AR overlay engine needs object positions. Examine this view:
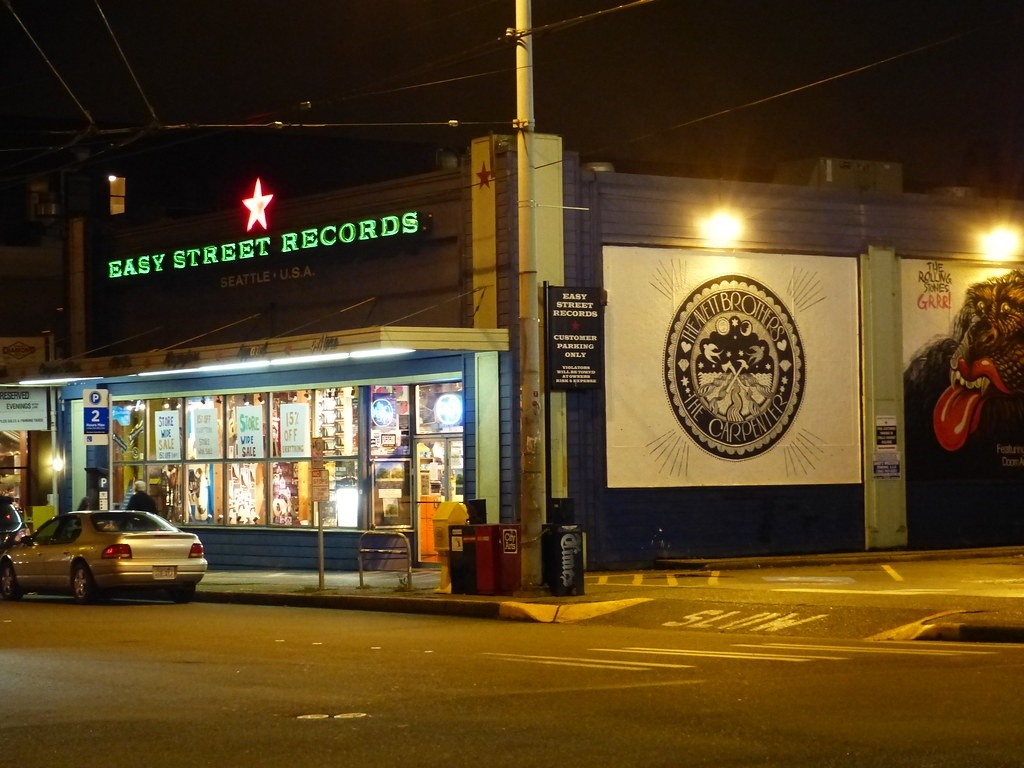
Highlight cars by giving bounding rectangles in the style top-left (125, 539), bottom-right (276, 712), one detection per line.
top-left (1, 510), bottom-right (208, 604)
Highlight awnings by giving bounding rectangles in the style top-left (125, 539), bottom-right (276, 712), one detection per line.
top-left (0, 325), bottom-right (508, 386)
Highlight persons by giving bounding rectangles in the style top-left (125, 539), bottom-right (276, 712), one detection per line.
top-left (120, 481), bottom-right (159, 532)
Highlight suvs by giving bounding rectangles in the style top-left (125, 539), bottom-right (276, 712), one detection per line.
top-left (0, 495), bottom-right (31, 600)
